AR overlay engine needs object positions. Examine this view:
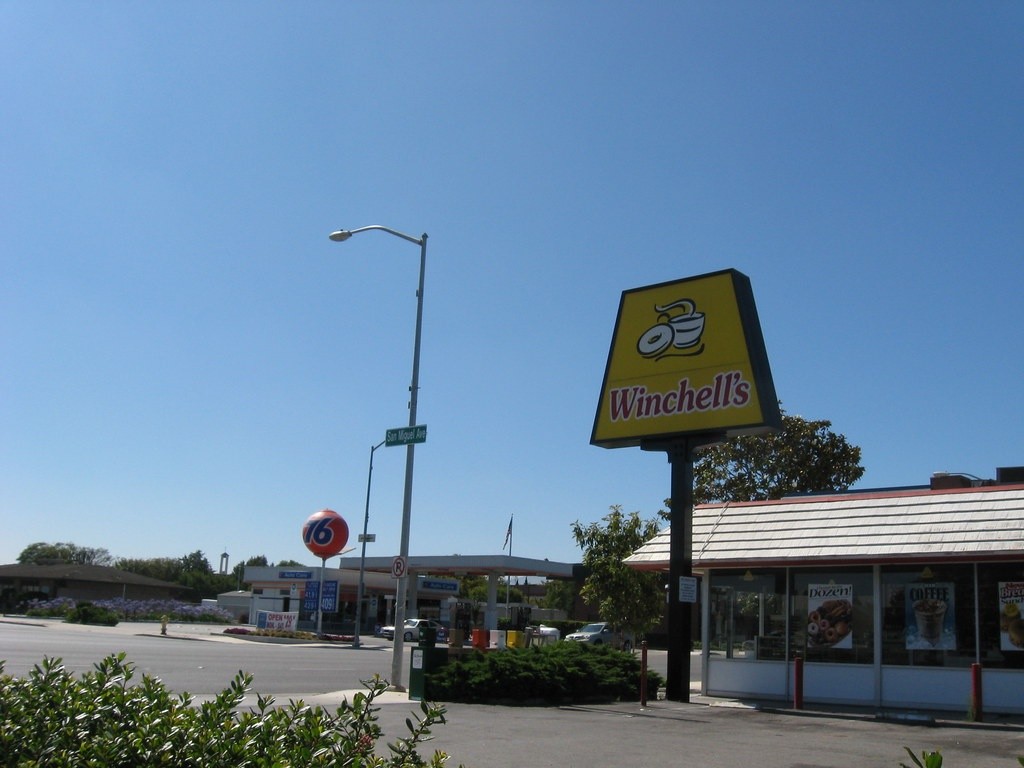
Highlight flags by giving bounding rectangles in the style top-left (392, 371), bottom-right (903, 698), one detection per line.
top-left (503, 516), bottom-right (512, 550)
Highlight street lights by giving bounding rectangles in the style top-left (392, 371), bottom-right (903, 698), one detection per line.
top-left (329, 227), bottom-right (431, 692)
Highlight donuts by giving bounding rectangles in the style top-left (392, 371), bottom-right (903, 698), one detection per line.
top-left (806, 610), bottom-right (838, 642)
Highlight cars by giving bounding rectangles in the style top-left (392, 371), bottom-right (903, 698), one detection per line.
top-left (379, 619), bottom-right (441, 643)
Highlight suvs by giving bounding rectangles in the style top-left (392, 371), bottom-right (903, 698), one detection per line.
top-left (564, 621), bottom-right (636, 654)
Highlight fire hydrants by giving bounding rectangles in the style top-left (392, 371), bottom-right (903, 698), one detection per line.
top-left (160, 615), bottom-right (169, 635)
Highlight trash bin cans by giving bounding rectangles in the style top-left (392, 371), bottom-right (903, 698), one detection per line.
top-left (472, 629), bottom-right (488, 649)
top-left (409, 645), bottom-right (449, 701)
top-left (448, 628), bottom-right (463, 647)
top-left (507, 630), bottom-right (526, 650)
top-left (418, 627), bottom-right (436, 647)
top-left (490, 630), bottom-right (506, 649)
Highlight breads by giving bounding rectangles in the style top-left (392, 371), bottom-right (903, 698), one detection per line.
top-left (1000, 602), bottom-right (1024, 648)
top-left (822, 601), bottom-right (849, 635)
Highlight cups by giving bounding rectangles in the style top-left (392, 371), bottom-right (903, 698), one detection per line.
top-left (913, 600), bottom-right (947, 646)
